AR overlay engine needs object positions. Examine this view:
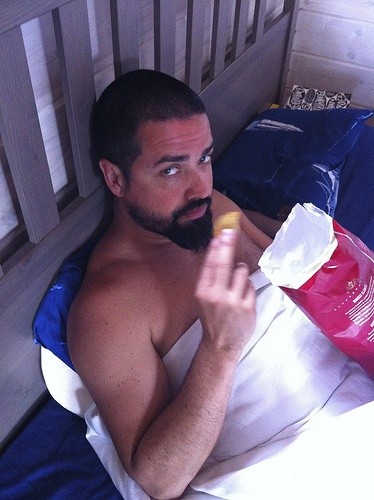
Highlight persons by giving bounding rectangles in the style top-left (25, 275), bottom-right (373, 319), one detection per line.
top-left (66, 67), bottom-right (374, 499)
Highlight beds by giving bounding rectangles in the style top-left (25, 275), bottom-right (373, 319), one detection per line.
top-left (0, 1), bottom-right (374, 499)
